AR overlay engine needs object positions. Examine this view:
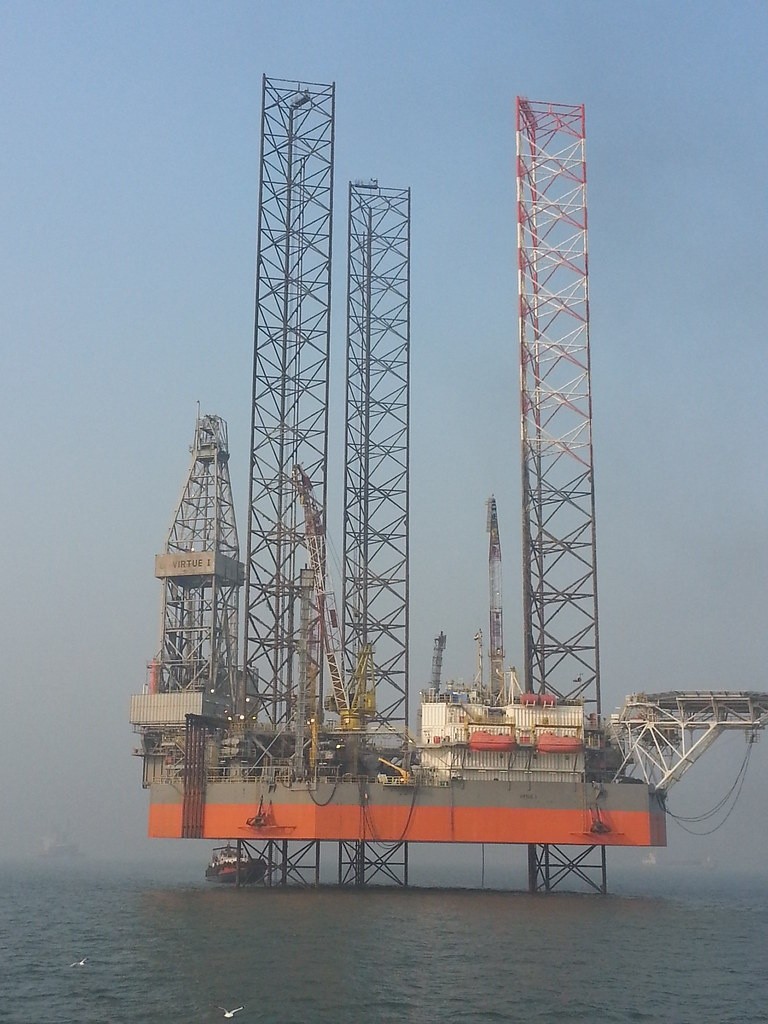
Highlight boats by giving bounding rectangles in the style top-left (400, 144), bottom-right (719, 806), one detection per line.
top-left (205, 841), bottom-right (269, 885)
